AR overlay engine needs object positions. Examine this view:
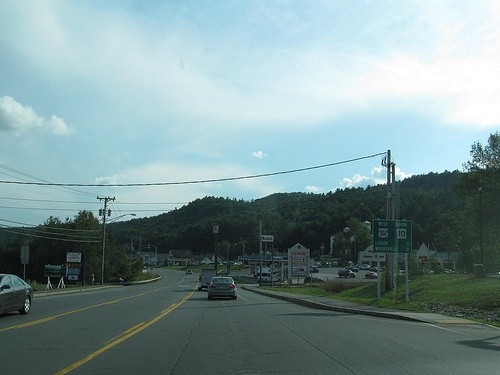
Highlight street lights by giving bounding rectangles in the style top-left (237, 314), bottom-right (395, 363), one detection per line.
top-left (101, 213), bottom-right (137, 284)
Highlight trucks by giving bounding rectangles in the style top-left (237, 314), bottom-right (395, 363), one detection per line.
top-left (338, 269), bottom-right (356, 278)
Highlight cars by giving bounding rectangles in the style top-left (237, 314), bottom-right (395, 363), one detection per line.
top-left (0, 274), bottom-right (33, 315)
top-left (198, 271), bottom-right (217, 291)
top-left (257, 273), bottom-right (280, 283)
top-left (365, 272), bottom-right (378, 279)
top-left (208, 276), bottom-right (237, 300)
